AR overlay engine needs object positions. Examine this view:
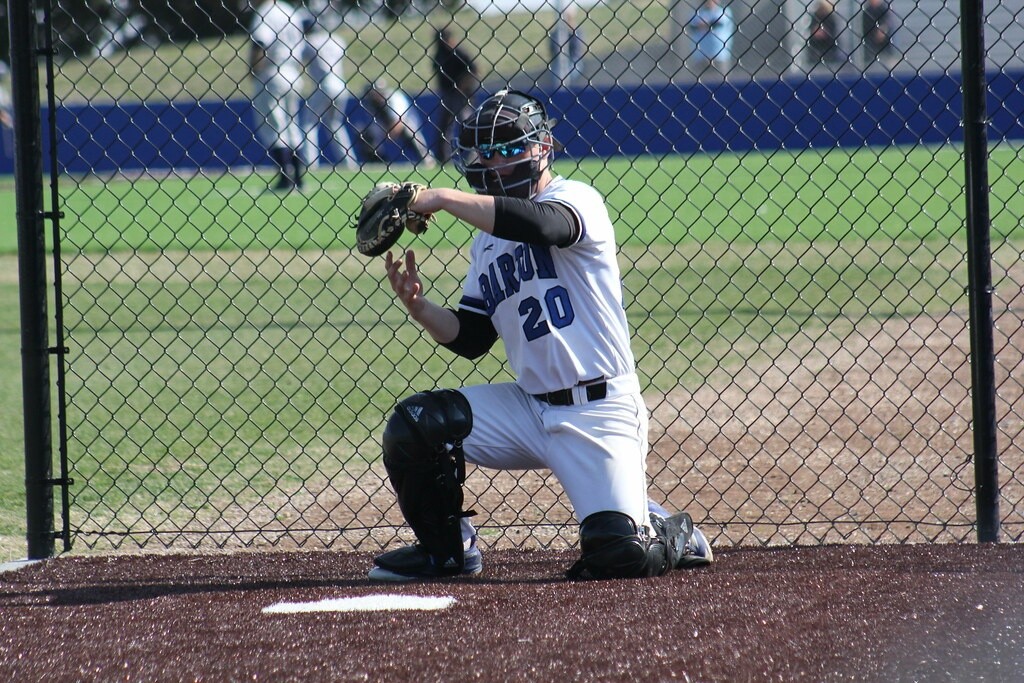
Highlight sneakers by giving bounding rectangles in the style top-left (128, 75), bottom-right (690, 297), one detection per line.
top-left (369, 544), bottom-right (483, 581)
top-left (647, 498), bottom-right (713, 568)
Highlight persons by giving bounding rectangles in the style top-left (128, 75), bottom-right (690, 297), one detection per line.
top-left (862, 0), bottom-right (901, 71)
top-left (299, 18), bottom-right (359, 171)
top-left (434, 27), bottom-right (480, 167)
top-left (549, 2), bottom-right (582, 87)
top-left (247, 0), bottom-right (307, 190)
top-left (688, 0), bottom-right (733, 82)
top-left (810, 0), bottom-right (843, 76)
top-left (363, 74), bottom-right (436, 170)
top-left (355, 89), bottom-right (712, 583)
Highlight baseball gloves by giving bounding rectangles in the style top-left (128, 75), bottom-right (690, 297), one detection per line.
top-left (348, 182), bottom-right (431, 257)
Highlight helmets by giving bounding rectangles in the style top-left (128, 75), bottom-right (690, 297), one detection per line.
top-left (456, 88), bottom-right (556, 203)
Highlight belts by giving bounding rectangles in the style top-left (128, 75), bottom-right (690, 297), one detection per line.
top-left (530, 382), bottom-right (608, 406)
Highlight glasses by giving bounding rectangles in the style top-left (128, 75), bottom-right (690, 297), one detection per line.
top-left (472, 136), bottom-right (535, 160)
